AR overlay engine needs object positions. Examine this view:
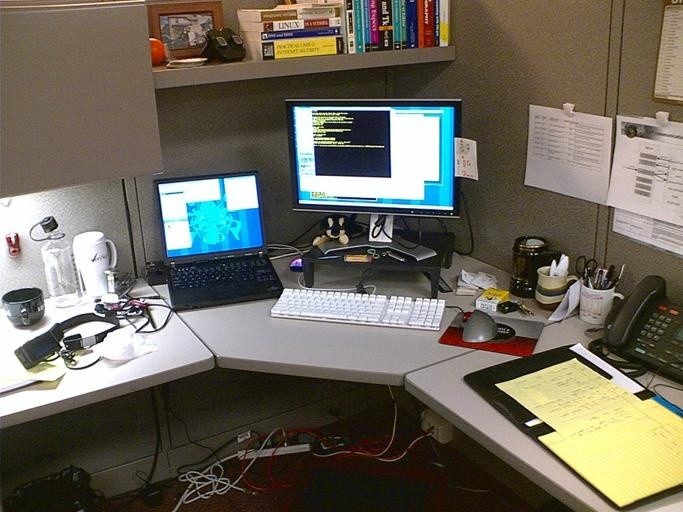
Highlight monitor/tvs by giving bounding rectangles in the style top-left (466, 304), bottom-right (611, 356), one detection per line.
top-left (285, 99), bottom-right (463, 262)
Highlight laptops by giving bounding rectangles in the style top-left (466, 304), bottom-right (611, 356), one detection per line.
top-left (153, 170), bottom-right (284, 311)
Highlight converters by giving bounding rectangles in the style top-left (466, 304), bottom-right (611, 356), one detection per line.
top-left (142, 484), bottom-right (163, 508)
top-left (420, 409), bottom-right (454, 444)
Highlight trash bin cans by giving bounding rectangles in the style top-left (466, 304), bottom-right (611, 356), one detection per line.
top-left (4, 474), bottom-right (91, 512)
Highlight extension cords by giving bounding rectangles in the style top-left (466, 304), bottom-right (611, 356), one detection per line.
top-left (237, 443), bottom-right (310, 460)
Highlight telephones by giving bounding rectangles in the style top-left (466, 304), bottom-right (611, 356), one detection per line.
top-left (605, 275), bottom-right (683, 385)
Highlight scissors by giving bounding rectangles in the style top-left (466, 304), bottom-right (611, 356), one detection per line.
top-left (576, 256), bottom-right (598, 280)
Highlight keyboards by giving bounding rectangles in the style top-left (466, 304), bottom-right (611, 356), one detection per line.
top-left (271, 289), bottom-right (446, 331)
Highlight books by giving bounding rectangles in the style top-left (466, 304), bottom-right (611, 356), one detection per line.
top-left (235, 0), bottom-right (448, 60)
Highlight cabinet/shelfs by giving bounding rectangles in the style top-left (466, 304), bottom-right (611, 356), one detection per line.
top-left (150, 43), bottom-right (459, 95)
top-left (5, 1), bottom-right (165, 204)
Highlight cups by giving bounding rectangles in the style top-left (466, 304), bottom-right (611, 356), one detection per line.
top-left (0, 287), bottom-right (44, 329)
top-left (535, 266), bottom-right (579, 311)
top-left (580, 279), bottom-right (626, 325)
top-left (72, 231), bottom-right (118, 295)
top-left (509, 236), bottom-right (548, 299)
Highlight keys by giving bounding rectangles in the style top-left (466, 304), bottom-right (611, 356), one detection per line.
top-left (497, 300), bottom-right (534, 318)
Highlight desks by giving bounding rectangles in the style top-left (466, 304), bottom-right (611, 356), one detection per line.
top-left (141, 234), bottom-right (584, 394)
top-left (0, 275), bottom-right (215, 430)
top-left (425, 312), bottom-right (682, 511)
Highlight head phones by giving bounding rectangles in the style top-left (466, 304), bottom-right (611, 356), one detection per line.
top-left (51, 312), bottom-right (119, 350)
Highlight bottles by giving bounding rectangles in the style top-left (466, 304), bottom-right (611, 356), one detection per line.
top-left (41, 229), bottom-right (84, 309)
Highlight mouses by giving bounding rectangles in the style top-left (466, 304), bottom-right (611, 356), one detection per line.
top-left (462, 310), bottom-right (498, 343)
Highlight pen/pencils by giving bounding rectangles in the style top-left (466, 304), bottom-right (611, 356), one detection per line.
top-left (585, 264), bottom-right (625, 290)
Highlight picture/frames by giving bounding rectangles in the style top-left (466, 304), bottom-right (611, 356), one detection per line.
top-left (145, 1), bottom-right (227, 62)
